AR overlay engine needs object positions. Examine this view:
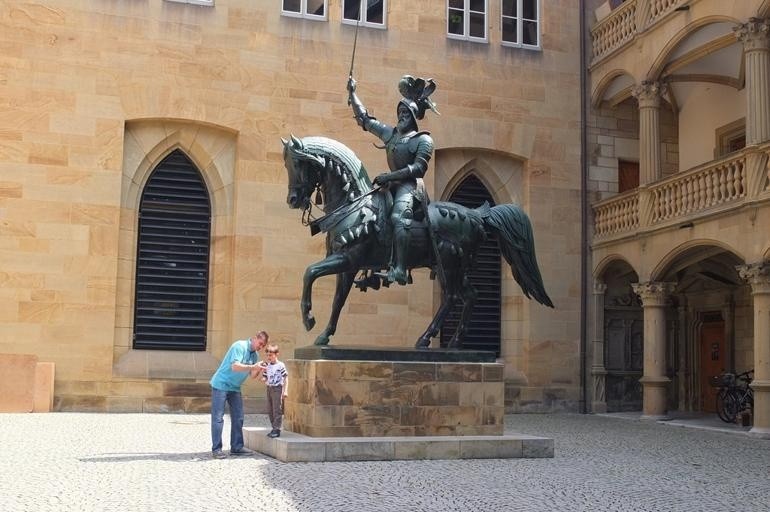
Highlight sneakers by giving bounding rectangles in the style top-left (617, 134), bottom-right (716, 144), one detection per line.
top-left (229, 447), bottom-right (255, 456)
top-left (212, 448), bottom-right (227, 459)
top-left (266, 429), bottom-right (281, 437)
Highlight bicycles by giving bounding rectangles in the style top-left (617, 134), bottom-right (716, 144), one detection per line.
top-left (713, 368), bottom-right (754, 425)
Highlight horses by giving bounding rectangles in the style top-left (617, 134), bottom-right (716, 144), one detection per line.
top-left (278, 134), bottom-right (556, 351)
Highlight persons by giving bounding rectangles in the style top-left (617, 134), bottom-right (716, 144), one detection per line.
top-left (347, 78), bottom-right (433, 290)
top-left (257, 344), bottom-right (288, 438)
top-left (209, 331), bottom-right (269, 459)
